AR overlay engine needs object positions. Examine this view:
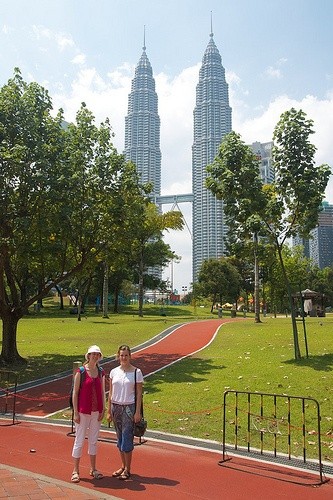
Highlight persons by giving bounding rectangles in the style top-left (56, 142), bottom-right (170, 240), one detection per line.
top-left (71, 345), bottom-right (106, 482)
top-left (107, 344), bottom-right (144, 480)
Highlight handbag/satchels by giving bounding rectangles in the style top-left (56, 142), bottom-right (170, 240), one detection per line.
top-left (133, 418), bottom-right (147, 437)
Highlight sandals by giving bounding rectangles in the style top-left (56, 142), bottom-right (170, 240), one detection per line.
top-left (71, 472), bottom-right (79, 482)
top-left (112, 468), bottom-right (130, 479)
top-left (90, 470), bottom-right (104, 479)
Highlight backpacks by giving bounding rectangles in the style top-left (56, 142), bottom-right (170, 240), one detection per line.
top-left (69, 388), bottom-right (73, 408)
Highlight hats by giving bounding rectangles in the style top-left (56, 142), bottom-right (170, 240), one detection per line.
top-left (85, 345), bottom-right (103, 360)
